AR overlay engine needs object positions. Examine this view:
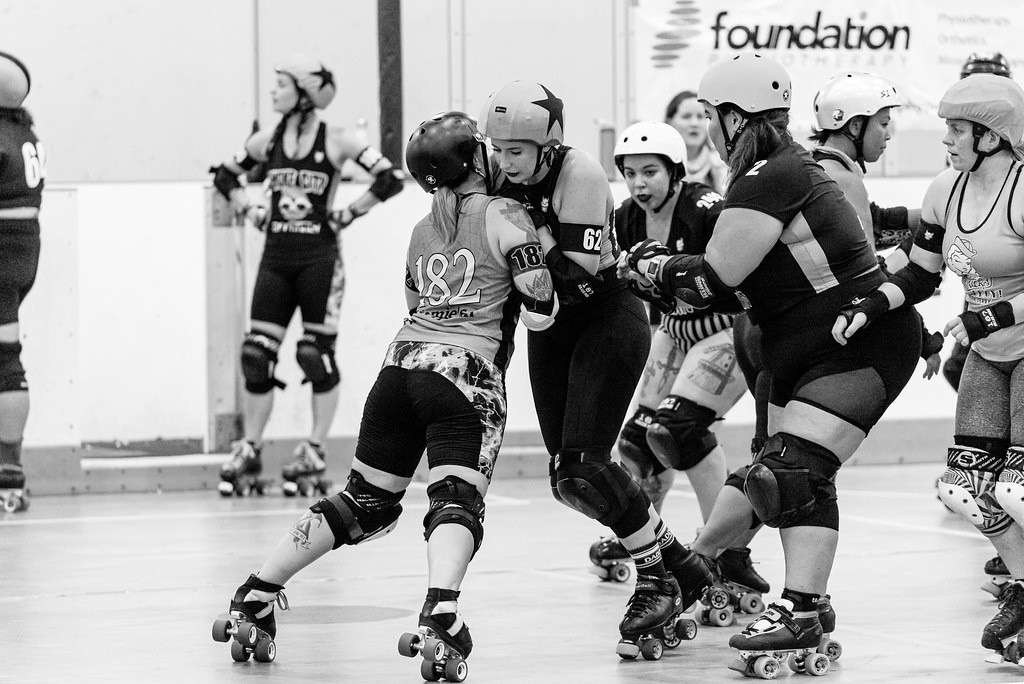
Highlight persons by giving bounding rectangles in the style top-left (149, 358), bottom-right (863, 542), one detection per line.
top-left (615, 51), bottom-right (925, 653)
top-left (587, 121), bottom-right (725, 582)
top-left (207, 56), bottom-right (403, 482)
top-left (229, 109), bottom-right (560, 660)
top-left (0, 52), bottom-right (44, 513)
top-left (831, 51), bottom-right (1024, 666)
top-left (665, 91), bottom-right (735, 196)
top-left (479, 80), bottom-right (715, 637)
top-left (714, 70), bottom-right (944, 593)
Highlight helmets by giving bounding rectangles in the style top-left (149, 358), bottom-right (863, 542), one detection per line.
top-left (696, 52), bottom-right (792, 118)
top-left (937, 72), bottom-right (1024, 158)
top-left (0, 51), bottom-right (30, 109)
top-left (961, 50), bottom-right (1011, 75)
top-left (813, 71), bottom-right (901, 130)
top-left (405, 110), bottom-right (486, 193)
top-left (275, 53), bottom-right (337, 110)
top-left (613, 121), bottom-right (687, 181)
top-left (477, 81), bottom-right (566, 144)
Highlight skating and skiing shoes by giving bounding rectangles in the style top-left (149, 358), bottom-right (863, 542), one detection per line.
top-left (589, 536), bottom-right (635, 582)
top-left (745, 594), bottom-right (843, 664)
top-left (728, 587), bottom-right (831, 680)
top-left (211, 574), bottom-right (291, 663)
top-left (685, 532), bottom-right (770, 615)
top-left (0, 463), bottom-right (29, 512)
top-left (981, 580), bottom-right (1024, 667)
top-left (615, 573), bottom-right (697, 661)
top-left (667, 551), bottom-right (730, 617)
top-left (280, 439), bottom-right (334, 497)
top-left (397, 587), bottom-right (475, 682)
top-left (217, 438), bottom-right (273, 497)
top-left (980, 556), bottom-right (1013, 597)
